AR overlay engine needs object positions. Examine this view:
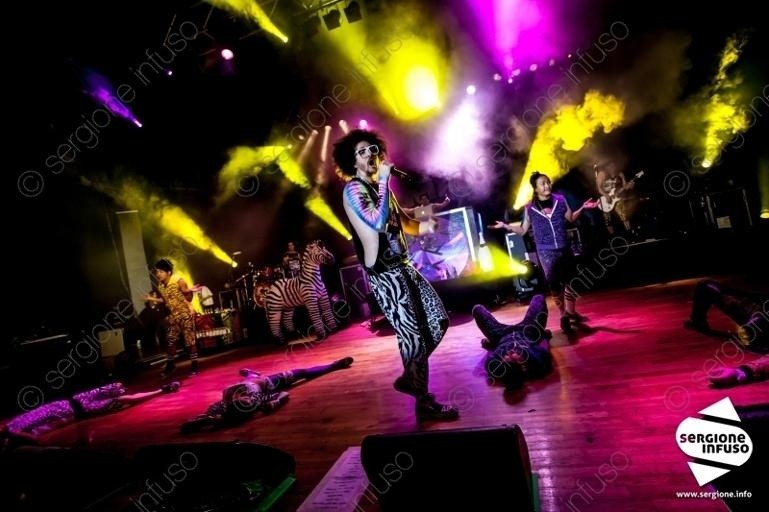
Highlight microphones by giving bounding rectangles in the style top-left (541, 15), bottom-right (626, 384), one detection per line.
top-left (390, 167), bottom-right (411, 181)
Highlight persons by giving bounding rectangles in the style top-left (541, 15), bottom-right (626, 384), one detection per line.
top-left (193, 280), bottom-right (215, 311)
top-left (141, 257), bottom-right (204, 375)
top-left (332, 126), bottom-right (460, 423)
top-left (178, 358), bottom-right (354, 435)
top-left (149, 291), bottom-right (163, 319)
top-left (1, 379), bottom-right (181, 449)
top-left (280, 240), bottom-right (301, 278)
top-left (684, 279), bottom-right (768, 388)
top-left (483, 171), bottom-right (603, 341)
top-left (399, 192), bottom-right (452, 235)
top-left (597, 161), bottom-right (639, 245)
top-left (470, 294), bottom-right (553, 394)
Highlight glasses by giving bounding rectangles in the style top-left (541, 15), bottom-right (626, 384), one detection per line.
top-left (354, 145), bottom-right (379, 158)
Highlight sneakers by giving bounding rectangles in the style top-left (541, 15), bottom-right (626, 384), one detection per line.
top-left (393, 376), bottom-right (433, 400)
top-left (415, 404), bottom-right (458, 421)
top-left (560, 317), bottom-right (570, 330)
top-left (161, 382), bottom-right (179, 392)
top-left (191, 360), bottom-right (198, 372)
top-left (240, 369), bottom-right (260, 376)
top-left (334, 357), bottom-right (353, 368)
top-left (565, 310), bottom-right (586, 321)
top-left (684, 320), bottom-right (706, 330)
top-left (162, 360), bottom-right (175, 374)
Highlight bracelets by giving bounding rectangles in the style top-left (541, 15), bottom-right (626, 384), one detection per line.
top-left (738, 363), bottom-right (756, 383)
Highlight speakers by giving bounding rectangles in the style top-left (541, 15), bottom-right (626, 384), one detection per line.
top-left (133, 444), bottom-right (296, 512)
top-left (361, 424), bottom-right (532, 512)
top-left (693, 405), bottom-right (769, 512)
